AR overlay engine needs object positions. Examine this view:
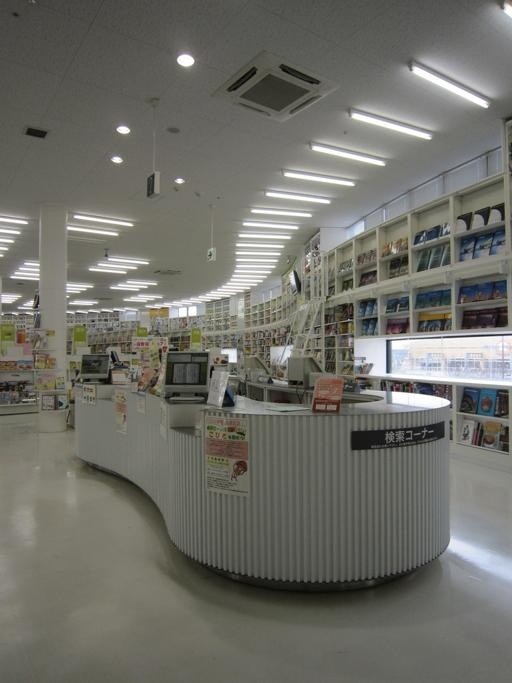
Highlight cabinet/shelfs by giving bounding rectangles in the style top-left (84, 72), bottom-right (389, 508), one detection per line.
top-left (0, 170), bottom-right (511, 452)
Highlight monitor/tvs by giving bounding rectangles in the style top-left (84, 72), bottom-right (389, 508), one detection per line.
top-left (80, 354), bottom-right (110, 384)
top-left (162, 351), bottom-right (211, 403)
top-left (288, 271), bottom-right (301, 296)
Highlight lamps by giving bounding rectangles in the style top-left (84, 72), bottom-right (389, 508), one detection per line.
top-left (346, 104), bottom-right (435, 141)
top-left (407, 55), bottom-right (494, 109)
top-left (309, 138), bottom-right (389, 166)
